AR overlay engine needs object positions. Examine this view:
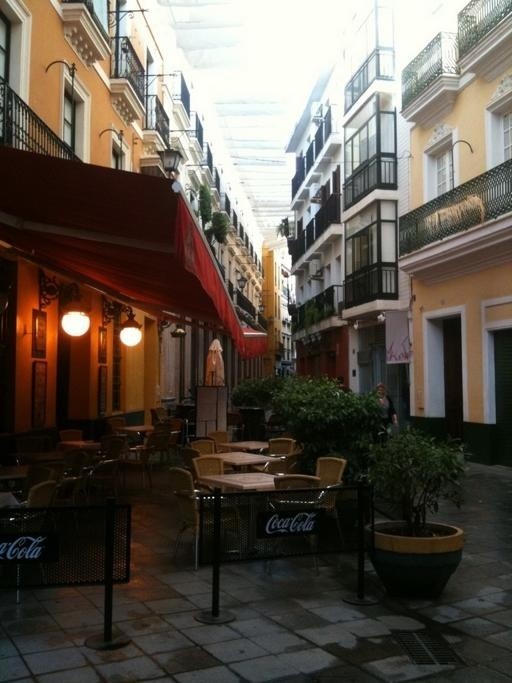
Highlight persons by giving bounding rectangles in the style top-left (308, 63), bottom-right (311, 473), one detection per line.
top-left (371, 380), bottom-right (400, 462)
top-left (336, 375), bottom-right (352, 396)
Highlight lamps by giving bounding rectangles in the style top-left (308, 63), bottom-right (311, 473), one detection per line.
top-left (158, 145), bottom-right (184, 180)
top-left (39, 275), bottom-right (143, 347)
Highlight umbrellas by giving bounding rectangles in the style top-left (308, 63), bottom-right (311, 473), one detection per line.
top-left (203, 338), bottom-right (227, 386)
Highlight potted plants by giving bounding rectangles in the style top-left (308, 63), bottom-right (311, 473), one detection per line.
top-left (353, 417), bottom-right (478, 601)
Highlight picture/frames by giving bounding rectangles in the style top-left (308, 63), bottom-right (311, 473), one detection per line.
top-left (96, 325), bottom-right (109, 416)
top-left (31, 308), bottom-right (49, 430)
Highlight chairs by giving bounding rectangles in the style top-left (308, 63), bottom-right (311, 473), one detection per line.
top-left (3, 406), bottom-right (350, 602)
